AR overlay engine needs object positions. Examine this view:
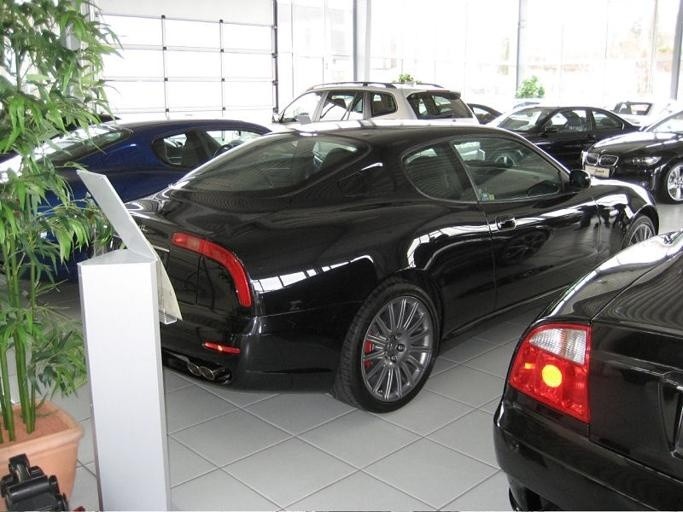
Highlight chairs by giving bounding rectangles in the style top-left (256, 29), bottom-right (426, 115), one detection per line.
top-left (153, 132), bottom-right (209, 166)
top-left (318, 146), bottom-right (463, 200)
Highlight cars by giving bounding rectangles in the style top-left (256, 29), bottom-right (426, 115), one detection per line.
top-left (416, 100), bottom-right (662, 165)
top-left (493, 228), bottom-right (683, 512)
top-left (82, 121), bottom-right (661, 414)
top-left (575, 109), bottom-right (683, 204)
top-left (0, 118), bottom-right (276, 285)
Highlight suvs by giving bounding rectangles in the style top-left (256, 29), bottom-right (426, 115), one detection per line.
top-left (228, 80), bottom-right (481, 150)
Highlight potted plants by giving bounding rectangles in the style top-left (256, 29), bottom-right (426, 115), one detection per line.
top-left (0, 0), bottom-right (135, 511)
